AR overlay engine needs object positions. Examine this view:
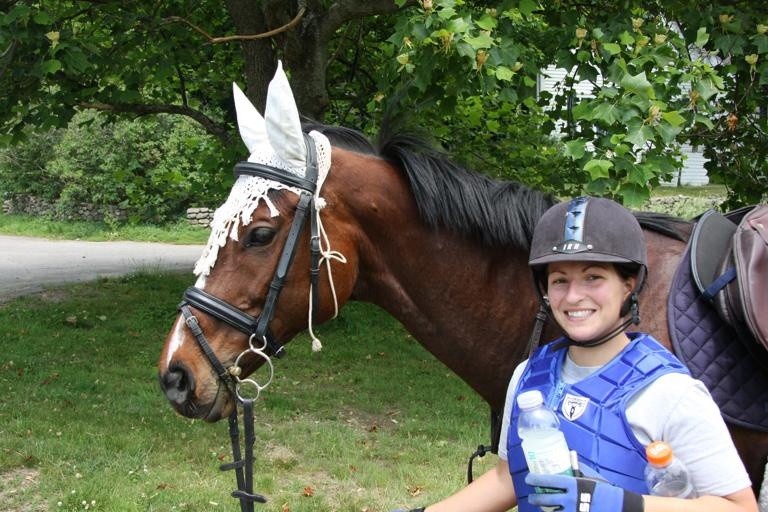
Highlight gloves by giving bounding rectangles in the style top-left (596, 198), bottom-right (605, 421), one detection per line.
top-left (525, 459), bottom-right (645, 512)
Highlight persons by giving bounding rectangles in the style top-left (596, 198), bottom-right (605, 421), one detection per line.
top-left (391, 194), bottom-right (760, 512)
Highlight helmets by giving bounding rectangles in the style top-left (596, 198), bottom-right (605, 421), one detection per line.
top-left (528, 196), bottom-right (647, 268)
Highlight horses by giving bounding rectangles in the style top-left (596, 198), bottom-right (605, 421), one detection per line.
top-left (155, 59), bottom-right (768, 508)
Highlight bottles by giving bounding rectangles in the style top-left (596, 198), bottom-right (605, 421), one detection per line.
top-left (515, 389), bottom-right (579, 511)
top-left (641, 439), bottom-right (698, 500)
